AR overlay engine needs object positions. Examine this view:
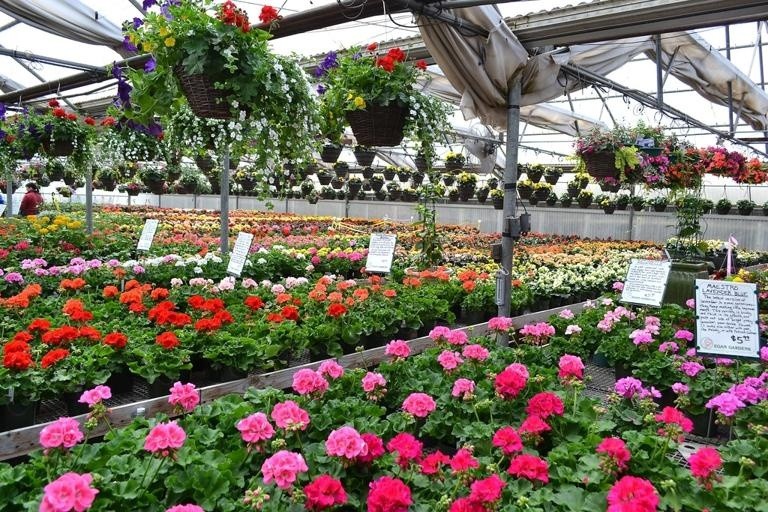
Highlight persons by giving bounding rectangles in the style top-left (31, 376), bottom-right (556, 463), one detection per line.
top-left (20, 182), bottom-right (43, 216)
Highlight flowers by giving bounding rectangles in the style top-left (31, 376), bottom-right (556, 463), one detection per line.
top-left (0, 0), bottom-right (768, 512)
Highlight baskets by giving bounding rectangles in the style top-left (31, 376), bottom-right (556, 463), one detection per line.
top-left (0, 127), bottom-right (768, 215)
top-left (171, 63), bottom-right (251, 120)
top-left (346, 100), bottom-right (411, 146)
